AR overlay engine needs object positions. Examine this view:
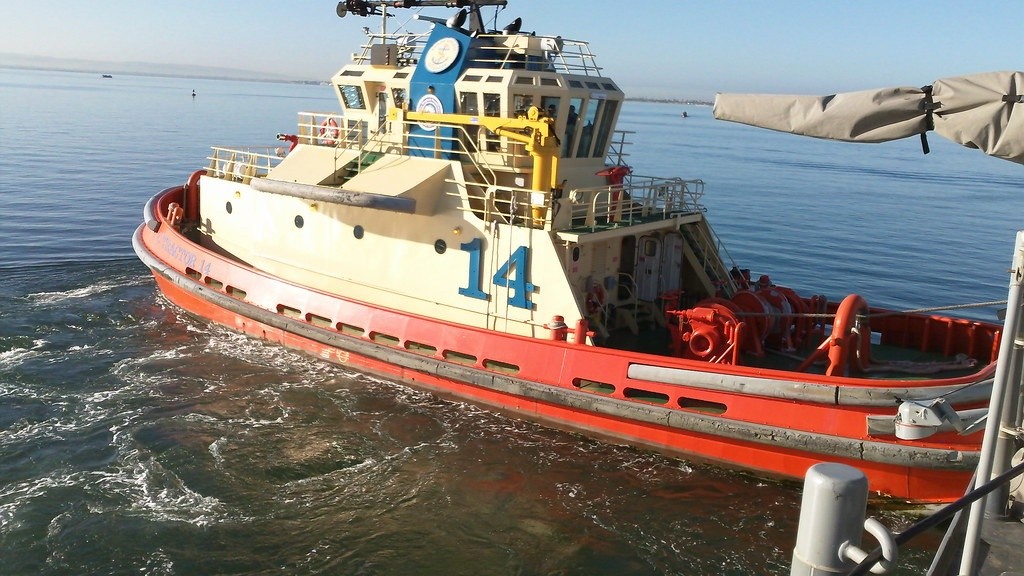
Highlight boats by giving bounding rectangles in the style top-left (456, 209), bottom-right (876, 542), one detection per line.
top-left (128, 2), bottom-right (1024, 504)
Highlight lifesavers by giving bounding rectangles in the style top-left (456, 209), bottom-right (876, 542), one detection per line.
top-left (586, 287), bottom-right (605, 314)
top-left (320, 119), bottom-right (339, 145)
